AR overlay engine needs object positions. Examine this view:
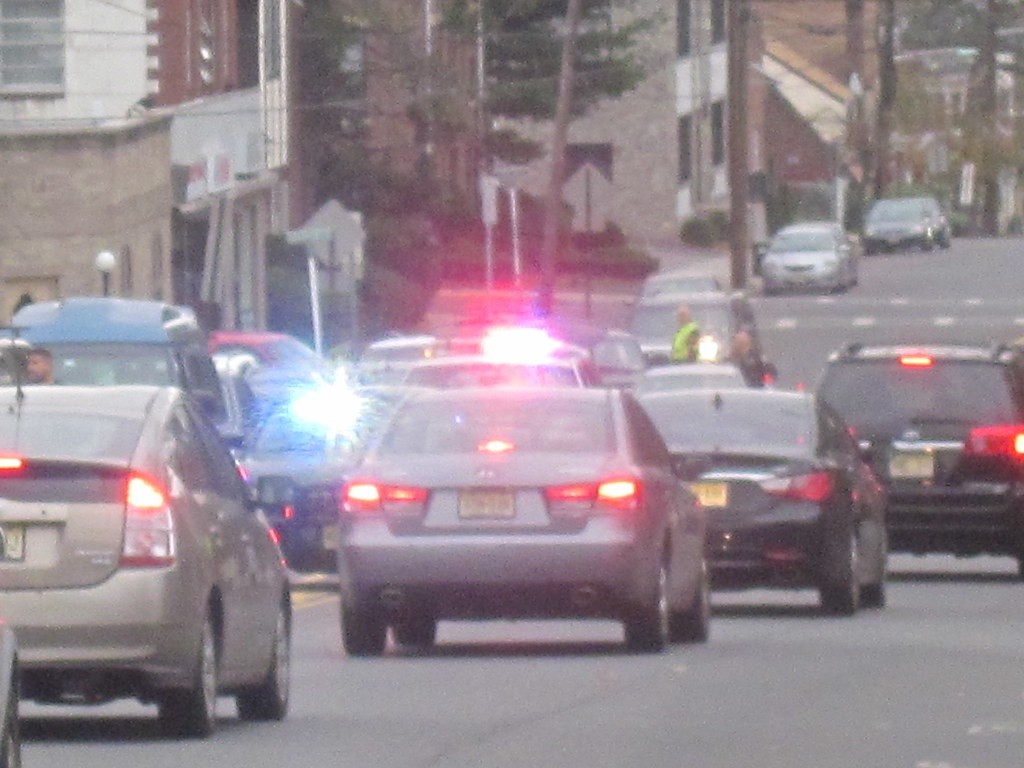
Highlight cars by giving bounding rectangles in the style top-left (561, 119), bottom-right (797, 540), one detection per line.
top-left (333, 384), bottom-right (715, 656)
top-left (752, 218), bottom-right (862, 295)
top-left (0, 376), bottom-right (301, 738)
top-left (640, 384), bottom-right (891, 619)
top-left (860, 193), bottom-right (952, 257)
top-left (205, 257), bottom-right (756, 568)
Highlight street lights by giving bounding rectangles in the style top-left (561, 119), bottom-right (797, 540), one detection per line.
top-left (93, 248), bottom-right (118, 297)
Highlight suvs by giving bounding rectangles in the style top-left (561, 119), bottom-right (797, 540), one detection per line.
top-left (817, 337), bottom-right (1024, 576)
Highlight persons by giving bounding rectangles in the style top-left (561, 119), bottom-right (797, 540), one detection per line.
top-left (730, 332), bottom-right (764, 388)
top-left (671, 307), bottom-right (701, 362)
top-left (23, 349), bottom-right (60, 386)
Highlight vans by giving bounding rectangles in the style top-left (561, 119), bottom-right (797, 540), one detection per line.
top-left (0, 293), bottom-right (226, 436)
